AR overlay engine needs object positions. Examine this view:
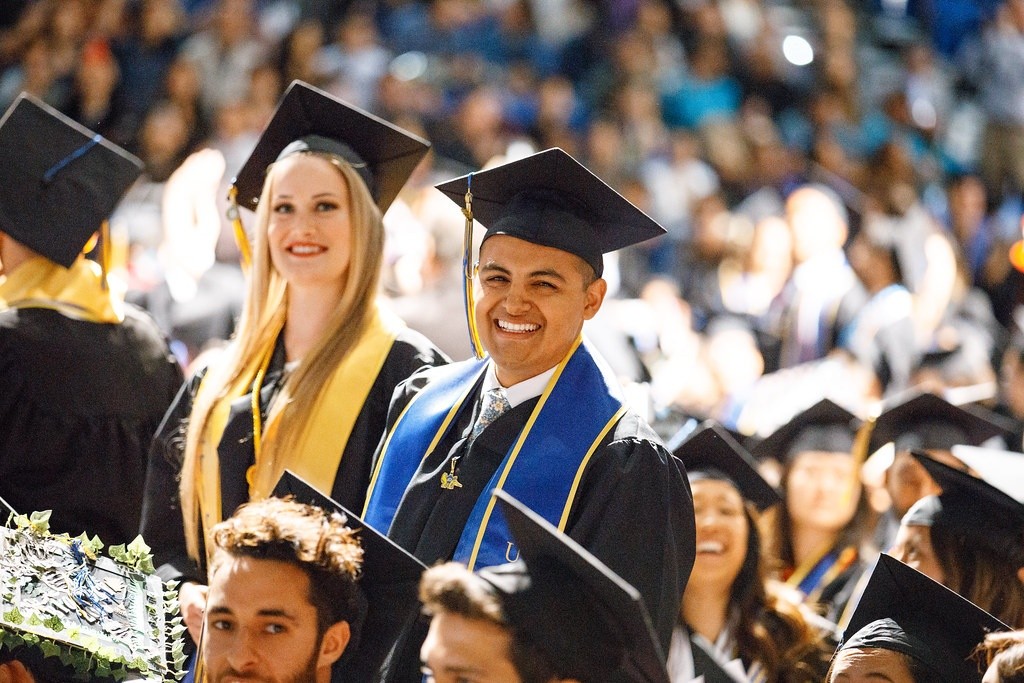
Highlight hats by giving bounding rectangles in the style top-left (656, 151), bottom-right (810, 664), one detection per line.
top-left (828, 553), bottom-right (1014, 683)
top-left (269, 470), bottom-right (429, 683)
top-left (226, 81), bottom-right (430, 275)
top-left (0, 92), bottom-right (145, 291)
top-left (900, 451), bottom-right (1024, 564)
top-left (672, 428), bottom-right (781, 512)
top-left (474, 491), bottom-right (670, 683)
top-left (749, 398), bottom-right (865, 452)
top-left (851, 392), bottom-right (1016, 455)
top-left (433, 147), bottom-right (668, 360)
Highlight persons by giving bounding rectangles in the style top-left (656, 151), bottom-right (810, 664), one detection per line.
top-left (0, 0), bottom-right (1024, 683)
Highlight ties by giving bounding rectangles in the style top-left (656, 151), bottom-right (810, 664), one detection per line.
top-left (462, 389), bottom-right (509, 459)
